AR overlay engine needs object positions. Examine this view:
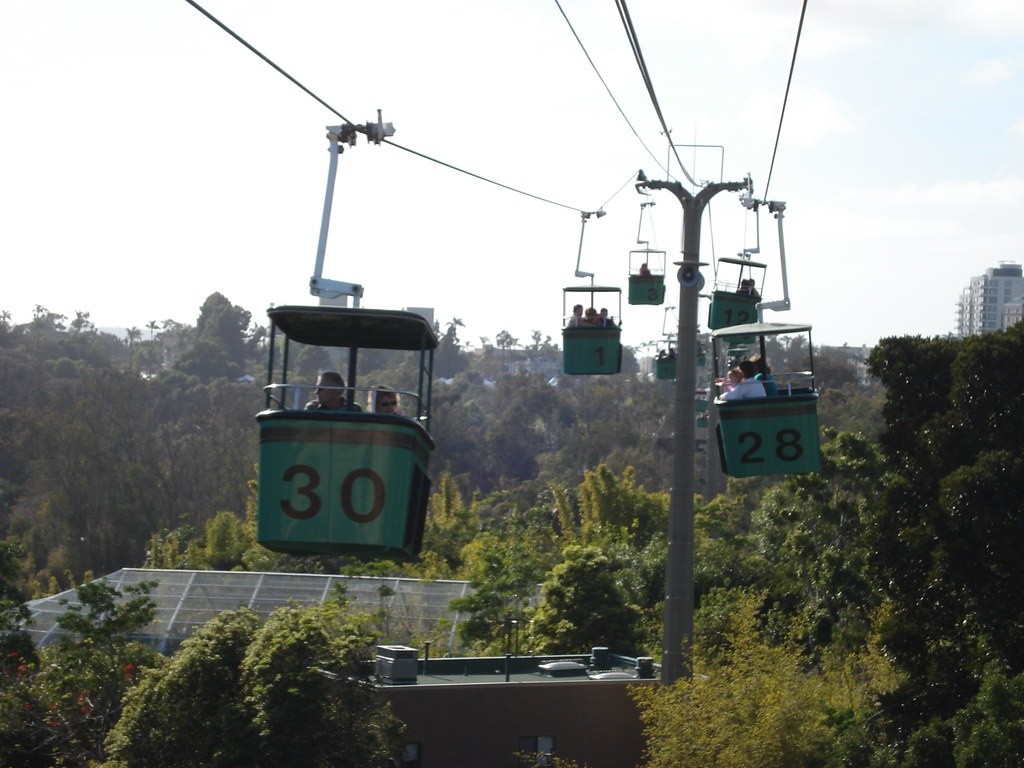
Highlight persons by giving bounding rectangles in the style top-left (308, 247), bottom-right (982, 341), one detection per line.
top-left (304, 371), bottom-right (363, 413)
top-left (657, 348), bottom-right (676, 361)
top-left (699, 352), bottom-right (779, 403)
top-left (639, 263), bottom-right (651, 276)
top-left (736, 279), bottom-right (759, 297)
top-left (568, 302), bottom-right (614, 327)
top-left (367, 383), bottom-right (411, 418)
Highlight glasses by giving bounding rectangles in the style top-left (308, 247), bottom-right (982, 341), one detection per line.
top-left (376, 401), bottom-right (397, 406)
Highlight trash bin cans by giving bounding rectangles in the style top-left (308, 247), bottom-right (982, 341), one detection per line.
top-left (374, 644), bottom-right (419, 685)
